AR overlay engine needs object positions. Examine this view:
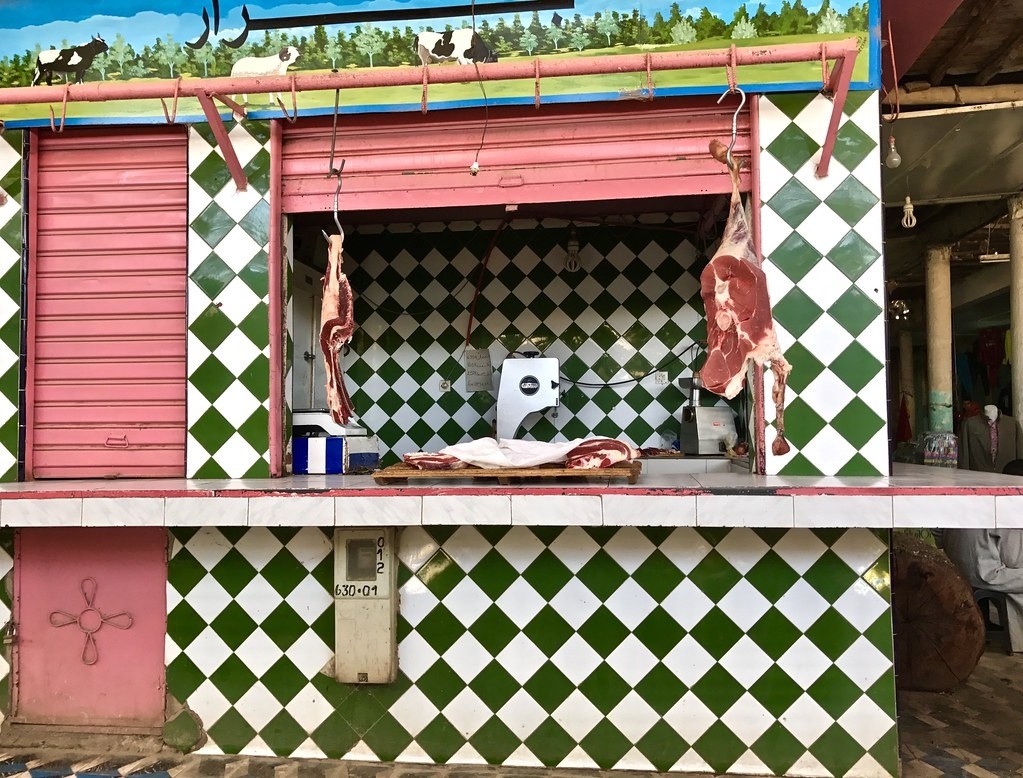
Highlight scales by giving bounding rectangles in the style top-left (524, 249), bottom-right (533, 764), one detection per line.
top-left (293, 408), bottom-right (368, 436)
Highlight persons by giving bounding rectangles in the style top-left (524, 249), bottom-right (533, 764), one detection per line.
top-left (956, 404), bottom-right (1023, 474)
top-left (943, 459), bottom-right (1023, 593)
